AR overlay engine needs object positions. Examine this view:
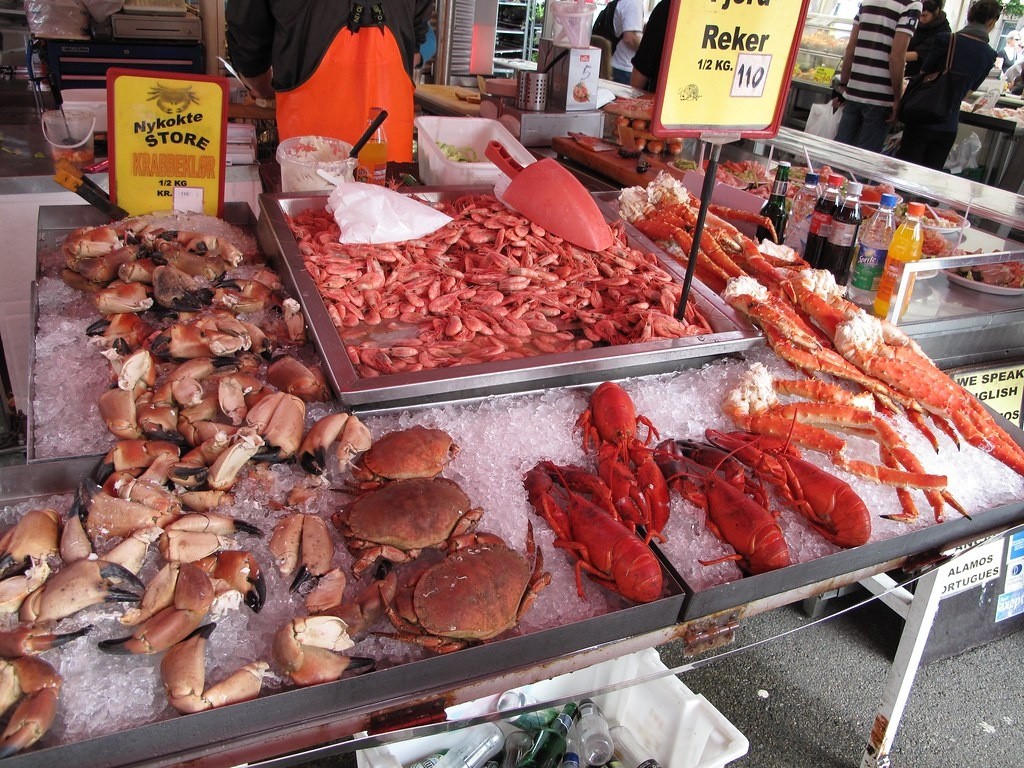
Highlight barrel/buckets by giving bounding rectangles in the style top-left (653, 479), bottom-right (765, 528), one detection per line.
top-left (41, 109), bottom-right (96, 163)
top-left (549, 1), bottom-right (598, 49)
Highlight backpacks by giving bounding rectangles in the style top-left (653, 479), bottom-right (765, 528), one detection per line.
top-left (592, 0), bottom-right (623, 57)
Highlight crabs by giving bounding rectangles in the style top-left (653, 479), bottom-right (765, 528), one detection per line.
top-left (619, 173), bottom-right (1023, 531)
top-left (334, 420), bottom-right (552, 654)
top-left (0, 217), bottom-right (372, 761)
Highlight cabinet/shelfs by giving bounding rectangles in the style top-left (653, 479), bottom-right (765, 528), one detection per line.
top-left (494, 0), bottom-right (531, 60)
top-left (792, 13), bottom-right (854, 88)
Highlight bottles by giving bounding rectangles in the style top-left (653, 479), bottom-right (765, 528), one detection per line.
top-left (802, 174), bottom-right (844, 270)
top-left (816, 182), bottom-right (863, 287)
top-left (755, 160), bottom-right (791, 246)
top-left (847, 192), bottom-right (898, 306)
top-left (408, 697), bottom-right (662, 767)
top-left (874, 202), bottom-right (926, 317)
top-left (356, 107), bottom-right (387, 188)
top-left (783, 172), bottom-right (820, 260)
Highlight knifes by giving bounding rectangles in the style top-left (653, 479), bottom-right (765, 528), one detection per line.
top-left (637, 152), bottom-right (648, 173)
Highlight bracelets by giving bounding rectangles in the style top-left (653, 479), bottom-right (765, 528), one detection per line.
top-left (838, 82), bottom-right (846, 87)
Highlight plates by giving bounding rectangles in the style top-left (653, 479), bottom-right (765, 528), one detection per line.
top-left (923, 206), bottom-right (970, 234)
top-left (916, 269), bottom-right (939, 283)
top-left (861, 185), bottom-right (903, 206)
top-left (940, 268), bottom-right (1024, 296)
top-left (667, 160), bottom-right (749, 190)
top-left (749, 181), bottom-right (771, 184)
top-left (832, 172), bottom-right (848, 183)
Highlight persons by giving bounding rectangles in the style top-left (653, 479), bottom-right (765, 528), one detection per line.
top-left (887, 0), bottom-right (1002, 170)
top-left (830, 0), bottom-right (923, 158)
top-left (905, 0), bottom-right (950, 79)
top-left (630, 0), bottom-right (672, 90)
top-left (226, 0), bottom-right (433, 164)
top-left (412, 23), bottom-right (437, 68)
top-left (997, 31), bottom-right (1024, 95)
top-left (599, 0), bottom-right (645, 85)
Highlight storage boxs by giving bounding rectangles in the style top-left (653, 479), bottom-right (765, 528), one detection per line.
top-left (412, 115), bottom-right (538, 186)
top-left (537, 38), bottom-right (602, 112)
top-left (502, 104), bottom-right (606, 146)
top-left (353, 647), bottom-right (749, 768)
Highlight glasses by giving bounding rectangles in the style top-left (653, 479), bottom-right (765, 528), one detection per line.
top-left (994, 5), bottom-right (1003, 18)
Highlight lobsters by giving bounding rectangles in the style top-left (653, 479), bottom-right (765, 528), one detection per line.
top-left (526, 379), bottom-right (871, 610)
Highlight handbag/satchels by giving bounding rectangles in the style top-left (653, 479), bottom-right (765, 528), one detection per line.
top-left (794, 98), bottom-right (845, 162)
top-left (881, 130), bottom-right (903, 158)
top-left (896, 32), bottom-right (964, 122)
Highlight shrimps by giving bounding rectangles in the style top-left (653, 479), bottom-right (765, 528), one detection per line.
top-left (286, 192), bottom-right (716, 377)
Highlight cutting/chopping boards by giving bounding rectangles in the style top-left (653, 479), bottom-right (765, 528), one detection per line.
top-left (551, 137), bottom-right (686, 190)
top-left (414, 84), bottom-right (481, 115)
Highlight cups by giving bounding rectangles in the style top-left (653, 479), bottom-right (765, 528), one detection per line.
top-left (276, 135), bottom-right (356, 192)
